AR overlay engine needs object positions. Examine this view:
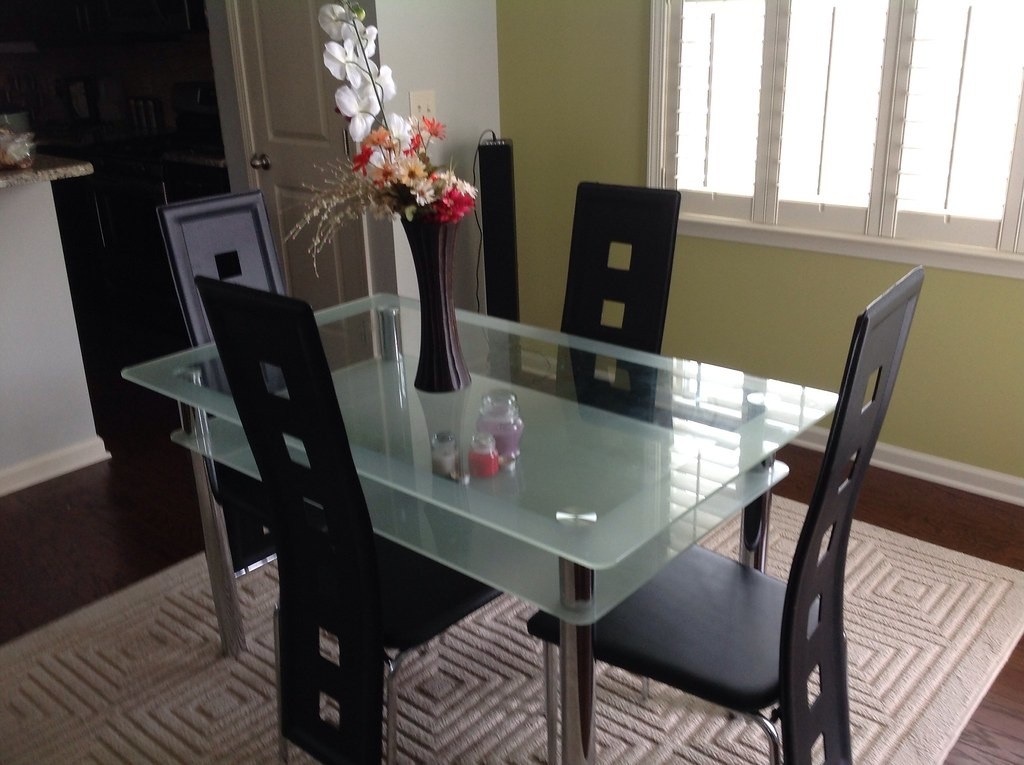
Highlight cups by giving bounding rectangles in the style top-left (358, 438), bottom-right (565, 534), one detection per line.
top-left (0, 110), bottom-right (31, 133)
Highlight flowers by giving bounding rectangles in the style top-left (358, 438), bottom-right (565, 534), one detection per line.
top-left (280, 0), bottom-right (479, 279)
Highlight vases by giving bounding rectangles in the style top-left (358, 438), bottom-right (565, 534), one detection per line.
top-left (400, 215), bottom-right (472, 393)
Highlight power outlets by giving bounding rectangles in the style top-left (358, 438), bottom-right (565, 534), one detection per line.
top-left (409, 90), bottom-right (435, 123)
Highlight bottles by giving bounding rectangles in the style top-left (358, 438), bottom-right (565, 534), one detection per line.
top-left (126, 85), bottom-right (164, 128)
top-left (468, 432), bottom-right (499, 478)
top-left (477, 390), bottom-right (524, 463)
top-left (430, 431), bottom-right (462, 481)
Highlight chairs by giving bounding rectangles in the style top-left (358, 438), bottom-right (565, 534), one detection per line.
top-left (153, 183), bottom-right (924, 765)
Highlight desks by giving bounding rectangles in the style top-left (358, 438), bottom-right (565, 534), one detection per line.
top-left (122, 293), bottom-right (839, 765)
